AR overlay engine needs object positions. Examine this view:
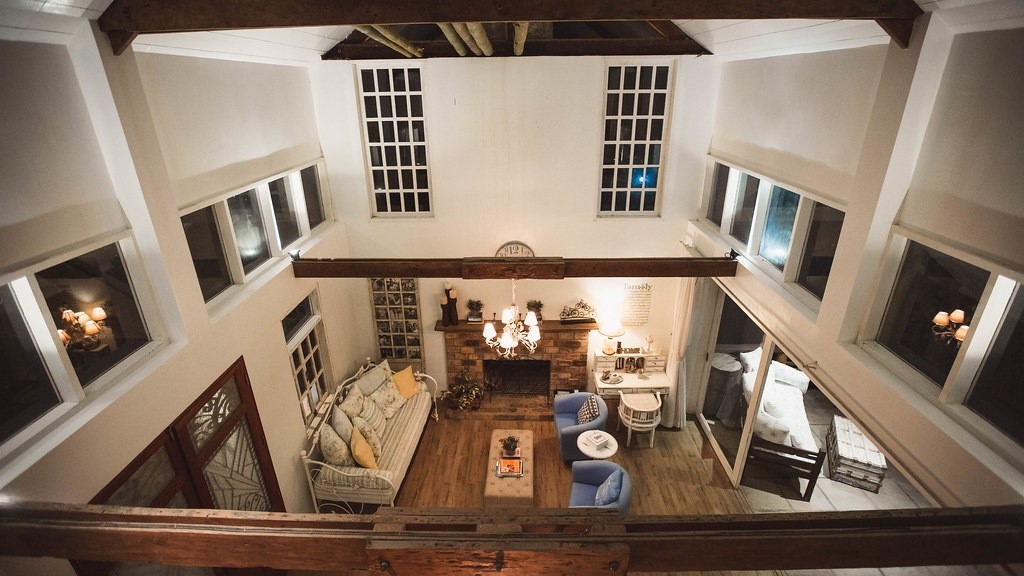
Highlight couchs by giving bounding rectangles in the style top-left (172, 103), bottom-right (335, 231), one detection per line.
top-left (552, 392), bottom-right (611, 461)
top-left (569, 461), bottom-right (632, 513)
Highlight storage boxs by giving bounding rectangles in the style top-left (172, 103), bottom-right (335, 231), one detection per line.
top-left (824, 414), bottom-right (889, 495)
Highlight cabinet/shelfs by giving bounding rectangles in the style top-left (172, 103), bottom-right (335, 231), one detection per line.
top-left (367, 277), bottom-right (427, 378)
top-left (592, 350), bottom-right (666, 372)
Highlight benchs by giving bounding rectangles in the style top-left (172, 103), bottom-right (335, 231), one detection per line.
top-left (301, 357), bottom-right (440, 514)
top-left (745, 360), bottom-right (827, 501)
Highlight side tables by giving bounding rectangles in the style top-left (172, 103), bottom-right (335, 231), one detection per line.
top-left (577, 429), bottom-right (618, 460)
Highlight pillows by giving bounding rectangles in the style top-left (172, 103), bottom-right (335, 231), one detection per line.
top-left (771, 359), bottom-right (811, 394)
top-left (751, 409), bottom-right (792, 447)
top-left (359, 396), bottom-right (386, 438)
top-left (351, 417), bottom-right (382, 457)
top-left (593, 467), bottom-right (622, 504)
top-left (361, 359), bottom-right (394, 379)
top-left (320, 423), bottom-right (355, 466)
top-left (389, 364), bottom-right (419, 399)
top-left (743, 372), bottom-right (784, 417)
top-left (338, 383), bottom-right (363, 419)
top-left (350, 426), bottom-right (377, 468)
top-left (740, 346), bottom-right (770, 376)
top-left (369, 380), bottom-right (406, 418)
top-left (332, 405), bottom-right (353, 445)
top-left (576, 393), bottom-right (599, 426)
top-left (358, 369), bottom-right (387, 396)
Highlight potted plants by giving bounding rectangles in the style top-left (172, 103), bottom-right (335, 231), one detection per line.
top-left (466, 298), bottom-right (484, 315)
top-left (443, 373), bottom-right (483, 410)
top-left (526, 300), bottom-right (543, 319)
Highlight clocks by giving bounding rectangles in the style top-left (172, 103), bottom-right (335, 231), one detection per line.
top-left (494, 239), bottom-right (536, 258)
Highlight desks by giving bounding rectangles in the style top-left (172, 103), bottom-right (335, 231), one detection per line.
top-left (593, 371), bottom-right (672, 432)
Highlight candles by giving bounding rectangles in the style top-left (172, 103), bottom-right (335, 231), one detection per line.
top-left (449, 289), bottom-right (457, 299)
top-left (444, 281), bottom-right (452, 289)
top-left (440, 297), bottom-right (447, 304)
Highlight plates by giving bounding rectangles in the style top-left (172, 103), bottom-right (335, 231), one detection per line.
top-left (600, 374), bottom-right (623, 384)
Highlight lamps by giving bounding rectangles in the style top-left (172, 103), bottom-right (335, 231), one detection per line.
top-left (929, 306), bottom-right (969, 345)
top-left (482, 279), bottom-right (542, 360)
top-left (56, 306), bottom-right (107, 349)
top-left (598, 315), bottom-right (626, 355)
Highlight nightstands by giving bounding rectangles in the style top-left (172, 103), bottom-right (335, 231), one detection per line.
top-left (704, 352), bottom-right (744, 428)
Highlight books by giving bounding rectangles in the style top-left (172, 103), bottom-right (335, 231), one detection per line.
top-left (496, 460), bottom-right (523, 476)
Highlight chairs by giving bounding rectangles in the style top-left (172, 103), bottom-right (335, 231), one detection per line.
top-left (616, 390), bottom-right (662, 450)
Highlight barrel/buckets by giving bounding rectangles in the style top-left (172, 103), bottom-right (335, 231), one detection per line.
top-left (703, 352), bottom-right (743, 417)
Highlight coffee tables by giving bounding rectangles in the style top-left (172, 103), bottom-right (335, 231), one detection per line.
top-left (483, 429), bottom-right (535, 507)
